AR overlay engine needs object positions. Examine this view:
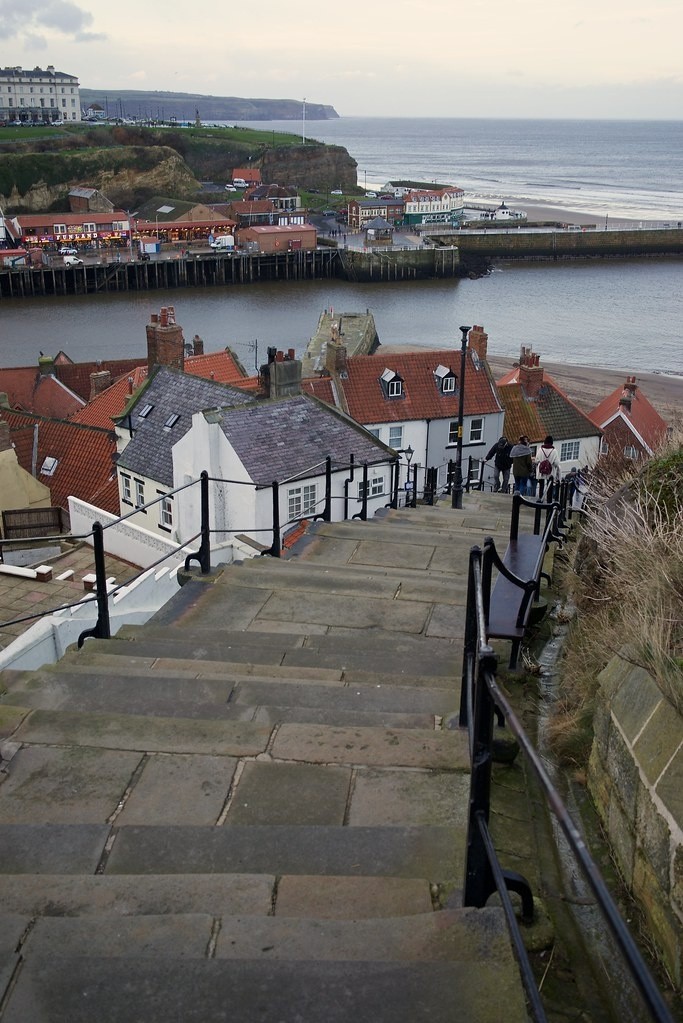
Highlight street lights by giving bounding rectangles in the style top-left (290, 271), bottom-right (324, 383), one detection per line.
top-left (404, 445), bottom-right (415, 503)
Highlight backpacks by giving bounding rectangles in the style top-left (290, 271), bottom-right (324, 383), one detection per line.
top-left (539, 447), bottom-right (554, 475)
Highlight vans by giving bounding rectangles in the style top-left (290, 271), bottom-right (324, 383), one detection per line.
top-left (63, 256), bottom-right (84, 267)
top-left (233, 178), bottom-right (249, 189)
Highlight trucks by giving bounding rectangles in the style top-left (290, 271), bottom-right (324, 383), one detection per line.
top-left (210, 235), bottom-right (234, 249)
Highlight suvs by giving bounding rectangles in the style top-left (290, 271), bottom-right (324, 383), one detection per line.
top-left (2, 119), bottom-right (64, 127)
top-left (331, 190), bottom-right (342, 196)
top-left (365, 192), bottom-right (393, 200)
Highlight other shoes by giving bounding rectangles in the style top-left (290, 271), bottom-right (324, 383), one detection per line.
top-left (493, 487), bottom-right (499, 492)
top-left (536, 499), bottom-right (543, 503)
top-left (501, 488), bottom-right (506, 493)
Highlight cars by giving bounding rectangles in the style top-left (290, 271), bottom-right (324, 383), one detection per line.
top-left (225, 185), bottom-right (236, 192)
top-left (58, 247), bottom-right (77, 255)
top-left (305, 188), bottom-right (319, 194)
top-left (322, 208), bottom-right (348, 216)
top-left (82, 114), bottom-right (101, 121)
top-left (138, 252), bottom-right (150, 261)
top-left (123, 119), bottom-right (135, 125)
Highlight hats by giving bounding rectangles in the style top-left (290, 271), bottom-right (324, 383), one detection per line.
top-left (571, 467), bottom-right (576, 472)
top-left (499, 437), bottom-right (507, 446)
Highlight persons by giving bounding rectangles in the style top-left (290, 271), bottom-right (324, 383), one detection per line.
top-left (484, 437), bottom-right (514, 494)
top-left (509, 435), bottom-right (560, 503)
